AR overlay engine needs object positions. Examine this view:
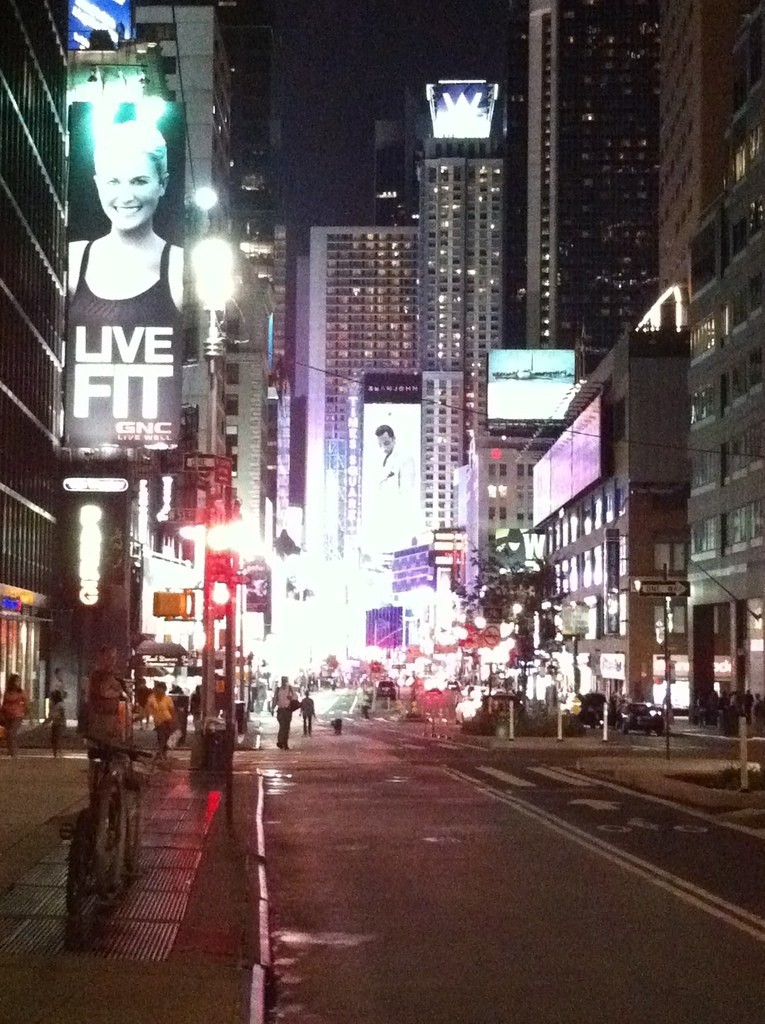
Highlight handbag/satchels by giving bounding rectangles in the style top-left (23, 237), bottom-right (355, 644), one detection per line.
top-left (290, 699), bottom-right (301, 712)
top-left (0, 706), bottom-right (9, 727)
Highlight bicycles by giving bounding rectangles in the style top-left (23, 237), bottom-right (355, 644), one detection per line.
top-left (57, 727), bottom-right (156, 948)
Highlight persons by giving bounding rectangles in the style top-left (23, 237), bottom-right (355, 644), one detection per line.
top-left (299, 690), bottom-right (315, 735)
top-left (695, 688), bottom-right (765, 727)
top-left (66, 120), bottom-right (183, 443)
top-left (132, 678), bottom-right (202, 759)
top-left (0, 674), bottom-right (29, 757)
top-left (271, 676), bottom-right (297, 750)
top-left (42, 689), bottom-right (65, 757)
top-left (374, 424), bottom-right (413, 550)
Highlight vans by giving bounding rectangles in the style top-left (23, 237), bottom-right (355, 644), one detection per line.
top-left (374, 680), bottom-right (397, 700)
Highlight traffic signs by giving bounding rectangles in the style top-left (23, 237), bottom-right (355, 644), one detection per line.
top-left (639, 580), bottom-right (691, 597)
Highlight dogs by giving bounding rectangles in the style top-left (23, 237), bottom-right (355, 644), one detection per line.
top-left (331, 718), bottom-right (342, 735)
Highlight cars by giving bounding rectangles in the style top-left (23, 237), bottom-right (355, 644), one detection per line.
top-left (582, 694), bottom-right (618, 726)
top-left (446, 679), bottom-right (526, 733)
top-left (615, 701), bottom-right (664, 734)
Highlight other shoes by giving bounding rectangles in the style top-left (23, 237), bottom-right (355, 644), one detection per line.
top-left (276, 743), bottom-right (288, 750)
